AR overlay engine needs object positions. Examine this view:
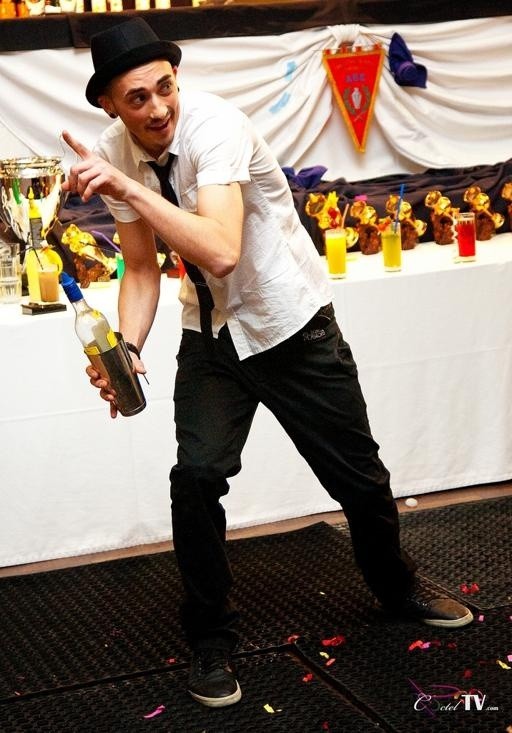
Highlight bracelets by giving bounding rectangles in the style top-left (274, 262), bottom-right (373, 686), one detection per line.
top-left (123, 340), bottom-right (140, 360)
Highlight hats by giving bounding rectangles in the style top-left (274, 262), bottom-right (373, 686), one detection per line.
top-left (85, 16), bottom-right (182, 108)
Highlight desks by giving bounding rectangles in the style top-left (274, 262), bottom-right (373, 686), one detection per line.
top-left (0, 230), bottom-right (512, 568)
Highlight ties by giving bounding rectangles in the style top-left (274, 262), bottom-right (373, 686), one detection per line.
top-left (143, 152), bottom-right (216, 351)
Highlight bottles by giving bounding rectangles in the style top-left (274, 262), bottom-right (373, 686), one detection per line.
top-left (60, 271), bottom-right (118, 354)
top-left (25, 206), bottom-right (57, 303)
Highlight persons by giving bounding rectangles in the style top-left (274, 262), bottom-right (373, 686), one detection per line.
top-left (59, 18), bottom-right (475, 707)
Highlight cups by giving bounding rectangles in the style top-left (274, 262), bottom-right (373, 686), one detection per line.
top-left (454, 212), bottom-right (479, 262)
top-left (326, 228), bottom-right (347, 280)
top-left (84, 330), bottom-right (147, 417)
top-left (0, 241), bottom-right (24, 304)
top-left (37, 263), bottom-right (58, 301)
top-left (378, 221), bottom-right (401, 272)
top-left (354, 215), bottom-right (496, 255)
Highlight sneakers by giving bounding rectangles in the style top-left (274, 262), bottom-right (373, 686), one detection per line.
top-left (385, 578), bottom-right (474, 628)
top-left (186, 638), bottom-right (243, 708)
